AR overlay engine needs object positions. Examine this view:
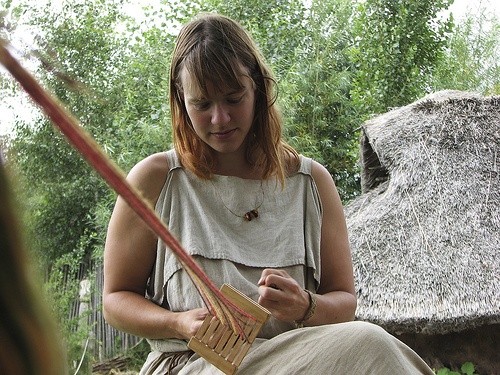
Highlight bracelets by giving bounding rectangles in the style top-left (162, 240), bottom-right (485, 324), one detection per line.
top-left (294, 290), bottom-right (318, 329)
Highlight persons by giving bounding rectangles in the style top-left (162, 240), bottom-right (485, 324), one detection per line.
top-left (103, 13), bottom-right (436, 374)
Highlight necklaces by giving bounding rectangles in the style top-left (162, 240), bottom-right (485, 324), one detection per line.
top-left (209, 172), bottom-right (266, 221)
